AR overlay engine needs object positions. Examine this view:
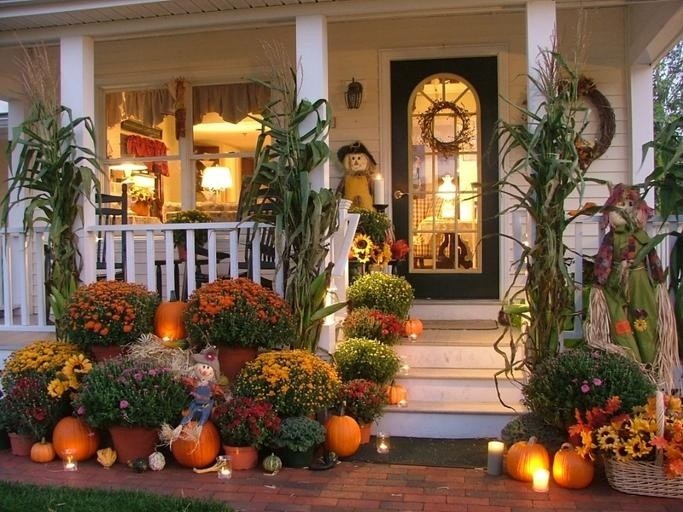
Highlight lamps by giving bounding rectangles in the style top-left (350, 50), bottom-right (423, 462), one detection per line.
top-left (343, 76), bottom-right (364, 108)
top-left (200, 153), bottom-right (233, 190)
top-left (108, 154), bottom-right (147, 172)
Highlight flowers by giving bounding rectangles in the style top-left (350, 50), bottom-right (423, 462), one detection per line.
top-left (1, 341), bottom-right (90, 432)
top-left (60, 281), bottom-right (153, 348)
top-left (571, 391), bottom-right (683, 477)
top-left (345, 234), bottom-right (407, 266)
top-left (523, 346), bottom-right (650, 426)
top-left (213, 349), bottom-right (339, 436)
top-left (70, 355), bottom-right (190, 432)
top-left (333, 270), bottom-right (414, 421)
top-left (187, 278), bottom-right (292, 346)
top-left (130, 186), bottom-right (154, 202)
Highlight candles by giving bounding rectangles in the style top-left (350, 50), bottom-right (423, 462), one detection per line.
top-left (485, 438), bottom-right (504, 478)
top-left (532, 469), bottom-right (546, 491)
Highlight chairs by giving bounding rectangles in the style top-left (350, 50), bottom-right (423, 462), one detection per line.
top-left (226, 207), bottom-right (288, 299)
top-left (41, 183), bottom-right (127, 324)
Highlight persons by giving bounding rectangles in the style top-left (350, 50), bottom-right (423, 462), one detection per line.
top-left (415, 154), bottom-right (422, 180)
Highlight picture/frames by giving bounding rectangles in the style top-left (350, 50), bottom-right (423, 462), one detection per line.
top-left (411, 144), bottom-right (426, 199)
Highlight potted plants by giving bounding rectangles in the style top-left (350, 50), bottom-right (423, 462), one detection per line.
top-left (162, 209), bottom-right (213, 261)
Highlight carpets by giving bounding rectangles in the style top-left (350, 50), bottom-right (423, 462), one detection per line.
top-left (413, 318), bottom-right (499, 332)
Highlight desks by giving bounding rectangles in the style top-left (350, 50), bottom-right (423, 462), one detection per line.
top-left (413, 225), bottom-right (480, 267)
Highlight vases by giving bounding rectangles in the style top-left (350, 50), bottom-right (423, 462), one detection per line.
top-left (107, 425), bottom-right (157, 463)
top-left (351, 419), bottom-right (371, 446)
top-left (224, 441), bottom-right (257, 470)
top-left (8, 432), bottom-right (38, 456)
top-left (135, 200), bottom-right (149, 216)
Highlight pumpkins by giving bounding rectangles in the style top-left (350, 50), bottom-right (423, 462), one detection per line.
top-left (399, 314), bottom-right (424, 338)
top-left (31, 437), bottom-right (56, 463)
top-left (506, 436), bottom-right (551, 482)
top-left (262, 452), bottom-right (283, 473)
top-left (552, 442), bottom-right (596, 489)
top-left (322, 408), bottom-right (362, 458)
top-left (148, 451), bottom-right (166, 471)
top-left (172, 416), bottom-right (221, 469)
top-left (383, 379), bottom-right (407, 405)
top-left (154, 290), bottom-right (188, 342)
top-left (96, 447), bottom-right (117, 468)
top-left (52, 411), bottom-right (103, 462)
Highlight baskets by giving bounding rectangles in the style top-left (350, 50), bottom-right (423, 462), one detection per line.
top-left (600, 392), bottom-right (683, 499)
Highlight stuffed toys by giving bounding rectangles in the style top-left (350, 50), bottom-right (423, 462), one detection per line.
top-left (591, 183), bottom-right (664, 364)
top-left (154, 344), bottom-right (220, 450)
top-left (334, 139), bottom-right (378, 213)
